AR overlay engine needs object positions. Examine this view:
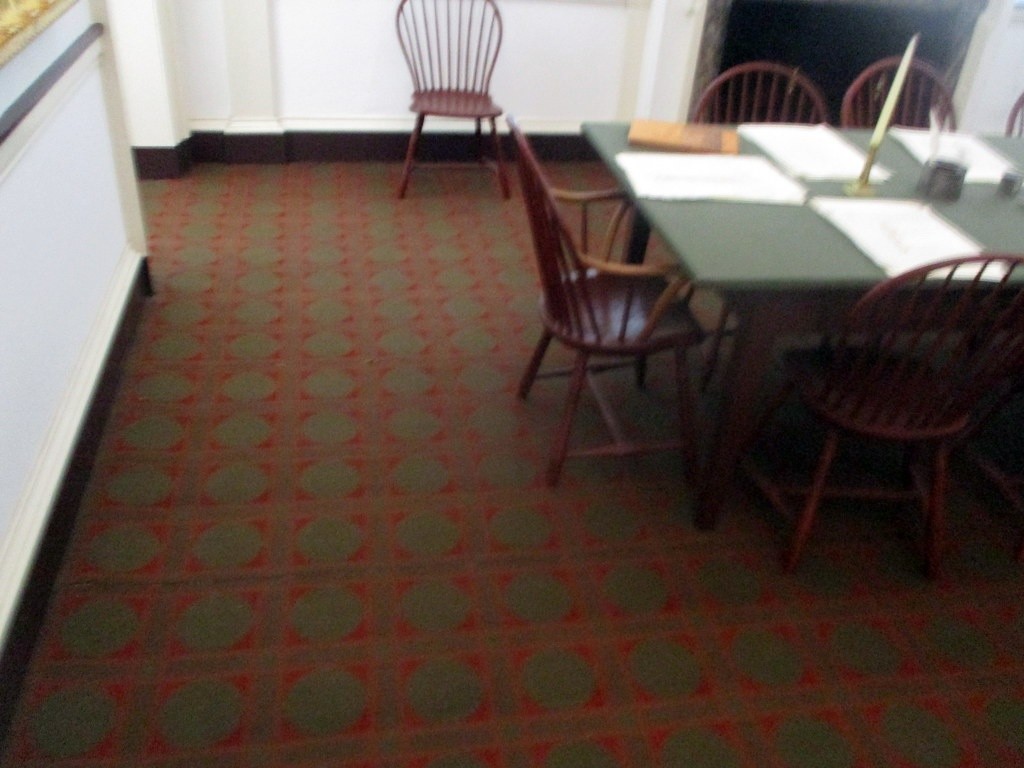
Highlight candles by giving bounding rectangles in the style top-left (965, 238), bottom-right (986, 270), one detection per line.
top-left (868, 32), bottom-right (923, 148)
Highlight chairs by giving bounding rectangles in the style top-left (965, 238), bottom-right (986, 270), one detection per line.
top-left (506, 57), bottom-right (1024, 580)
top-left (392, 0), bottom-right (513, 203)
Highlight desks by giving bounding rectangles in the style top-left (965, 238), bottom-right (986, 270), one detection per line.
top-left (577, 120), bottom-right (1024, 533)
